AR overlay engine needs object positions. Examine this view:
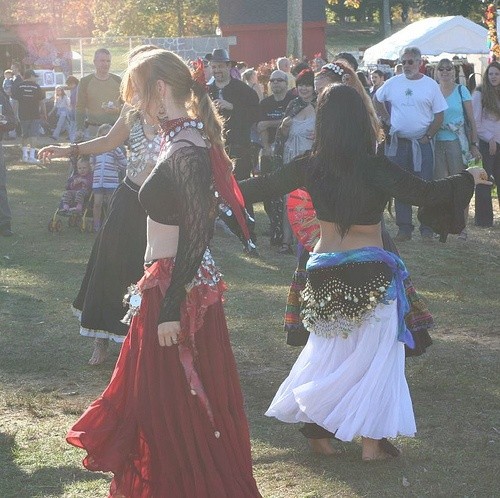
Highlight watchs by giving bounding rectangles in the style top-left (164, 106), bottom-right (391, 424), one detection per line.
top-left (470, 142), bottom-right (480, 147)
top-left (425, 134), bottom-right (432, 139)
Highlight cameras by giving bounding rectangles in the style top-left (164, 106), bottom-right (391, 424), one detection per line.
top-left (101, 101), bottom-right (114, 110)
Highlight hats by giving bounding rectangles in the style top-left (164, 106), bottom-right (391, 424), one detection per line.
top-left (24, 69), bottom-right (39, 77)
top-left (204, 48), bottom-right (233, 62)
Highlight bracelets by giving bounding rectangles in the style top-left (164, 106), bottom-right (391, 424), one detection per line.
top-left (70, 143), bottom-right (80, 160)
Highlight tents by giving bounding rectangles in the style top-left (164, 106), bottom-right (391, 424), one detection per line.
top-left (362, 15), bottom-right (491, 68)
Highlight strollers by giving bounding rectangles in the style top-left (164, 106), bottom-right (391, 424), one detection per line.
top-left (47, 131), bottom-right (106, 233)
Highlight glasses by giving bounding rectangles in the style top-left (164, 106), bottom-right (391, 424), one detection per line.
top-left (268, 78), bottom-right (288, 82)
top-left (439, 66), bottom-right (454, 71)
top-left (400, 59), bottom-right (413, 65)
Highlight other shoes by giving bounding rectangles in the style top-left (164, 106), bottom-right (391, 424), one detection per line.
top-left (1, 228), bottom-right (14, 236)
top-left (49, 135), bottom-right (58, 141)
top-left (22, 156), bottom-right (29, 163)
top-left (250, 233), bottom-right (290, 254)
top-left (28, 157), bottom-right (37, 164)
top-left (422, 234), bottom-right (433, 244)
top-left (393, 232), bottom-right (412, 241)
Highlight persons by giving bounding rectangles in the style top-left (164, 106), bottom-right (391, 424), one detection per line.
top-left (223, 84), bottom-right (493, 460)
top-left (0, 44), bottom-right (500, 365)
top-left (66, 50), bottom-right (264, 498)
